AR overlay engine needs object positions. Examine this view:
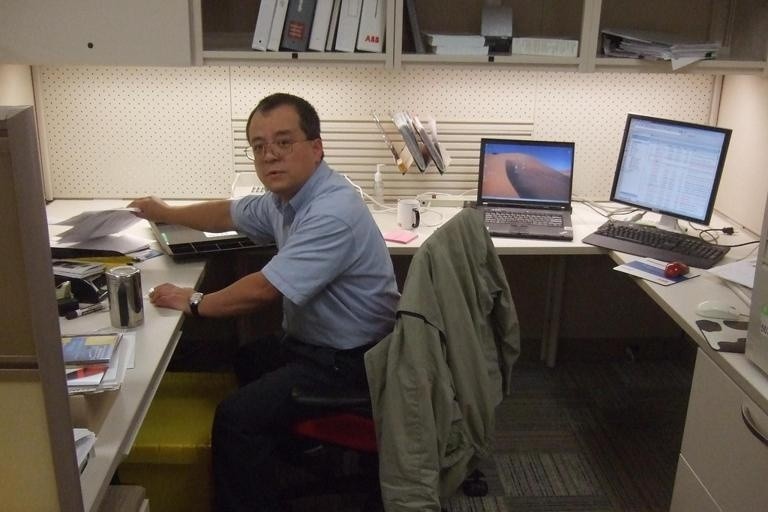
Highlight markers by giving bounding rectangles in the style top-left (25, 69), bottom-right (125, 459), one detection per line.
top-left (63, 301), bottom-right (114, 322)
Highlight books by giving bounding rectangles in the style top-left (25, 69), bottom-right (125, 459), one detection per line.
top-left (62, 333), bottom-right (118, 362)
top-left (63, 335), bottom-right (123, 364)
top-left (422, 30), bottom-right (486, 47)
top-left (406, 0), bottom-right (426, 54)
top-left (432, 46), bottom-right (489, 57)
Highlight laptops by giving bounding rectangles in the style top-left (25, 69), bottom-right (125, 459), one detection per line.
top-left (477, 138), bottom-right (575, 241)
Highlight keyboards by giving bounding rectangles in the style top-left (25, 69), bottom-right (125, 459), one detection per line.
top-left (584, 219), bottom-right (730, 268)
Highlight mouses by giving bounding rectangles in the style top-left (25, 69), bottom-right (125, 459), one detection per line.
top-left (698, 298), bottom-right (742, 324)
top-left (665, 261), bottom-right (690, 278)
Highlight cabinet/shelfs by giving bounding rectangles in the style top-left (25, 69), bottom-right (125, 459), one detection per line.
top-left (188, 0), bottom-right (768, 66)
top-left (671, 344), bottom-right (768, 512)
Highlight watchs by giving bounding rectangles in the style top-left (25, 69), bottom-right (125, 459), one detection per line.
top-left (191, 292), bottom-right (203, 321)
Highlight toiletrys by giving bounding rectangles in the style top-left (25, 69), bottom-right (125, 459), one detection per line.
top-left (373, 163), bottom-right (386, 211)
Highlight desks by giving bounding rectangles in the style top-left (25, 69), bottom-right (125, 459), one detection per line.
top-left (45, 197), bottom-right (226, 512)
top-left (620, 196), bottom-right (758, 346)
top-left (233, 189), bottom-right (728, 368)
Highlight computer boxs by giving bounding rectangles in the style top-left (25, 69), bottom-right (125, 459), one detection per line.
top-left (744, 199), bottom-right (768, 380)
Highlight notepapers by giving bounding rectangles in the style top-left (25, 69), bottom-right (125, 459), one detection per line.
top-left (380, 228), bottom-right (420, 244)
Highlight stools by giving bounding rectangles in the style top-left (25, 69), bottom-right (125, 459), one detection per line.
top-left (286, 377), bottom-right (378, 454)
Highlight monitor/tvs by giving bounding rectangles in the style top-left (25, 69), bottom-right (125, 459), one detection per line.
top-left (609, 112), bottom-right (734, 225)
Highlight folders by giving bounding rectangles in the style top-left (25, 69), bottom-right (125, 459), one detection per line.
top-left (412, 119), bottom-right (463, 176)
top-left (601, 26), bottom-right (670, 62)
top-left (251, 1), bottom-right (385, 52)
top-left (384, 112), bottom-right (431, 172)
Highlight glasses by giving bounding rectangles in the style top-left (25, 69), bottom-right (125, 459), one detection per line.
top-left (245, 136), bottom-right (309, 161)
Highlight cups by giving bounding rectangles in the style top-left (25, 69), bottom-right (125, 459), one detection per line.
top-left (395, 199), bottom-right (421, 232)
top-left (105, 265), bottom-right (146, 329)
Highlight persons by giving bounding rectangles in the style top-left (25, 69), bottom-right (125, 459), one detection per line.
top-left (130, 93), bottom-right (402, 512)
